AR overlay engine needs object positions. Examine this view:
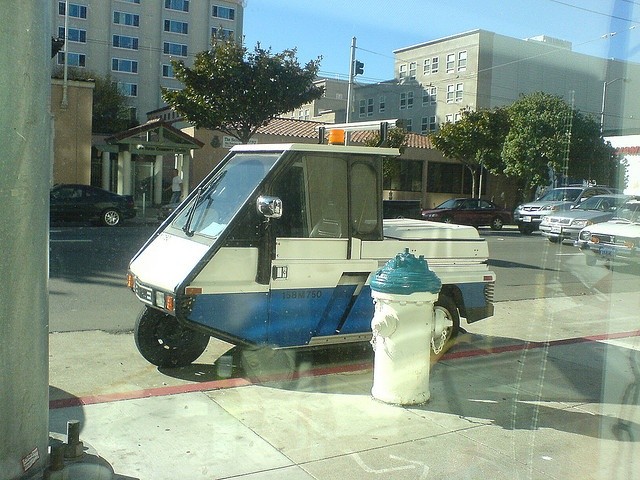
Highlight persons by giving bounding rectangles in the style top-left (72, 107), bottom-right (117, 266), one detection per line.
top-left (164, 168), bottom-right (183, 204)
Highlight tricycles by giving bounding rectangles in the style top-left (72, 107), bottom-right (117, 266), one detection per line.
top-left (127, 119), bottom-right (497, 366)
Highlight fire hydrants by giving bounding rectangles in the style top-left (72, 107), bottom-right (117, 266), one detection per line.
top-left (370, 248), bottom-right (454, 405)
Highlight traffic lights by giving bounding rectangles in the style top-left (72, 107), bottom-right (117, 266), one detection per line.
top-left (356, 60), bottom-right (364, 74)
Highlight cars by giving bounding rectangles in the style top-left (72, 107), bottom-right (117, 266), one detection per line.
top-left (514, 187), bottom-right (614, 235)
top-left (422, 198), bottom-right (511, 230)
top-left (539, 194), bottom-right (640, 243)
top-left (578, 199), bottom-right (640, 266)
top-left (50, 184), bottom-right (136, 226)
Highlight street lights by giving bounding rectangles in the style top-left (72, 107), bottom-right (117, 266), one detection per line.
top-left (600, 77), bottom-right (632, 137)
